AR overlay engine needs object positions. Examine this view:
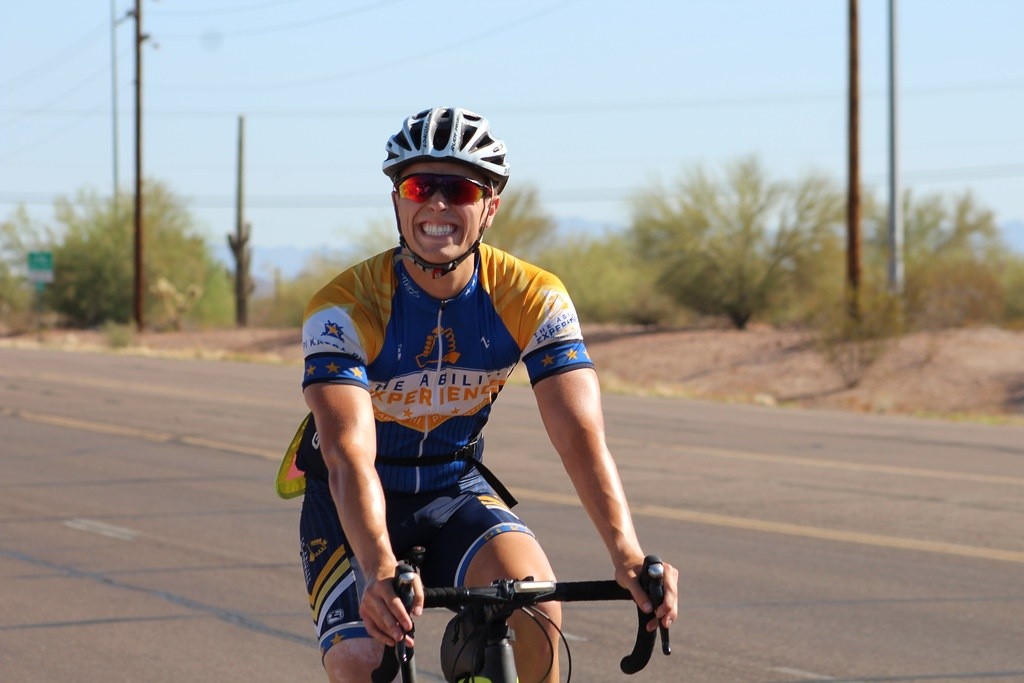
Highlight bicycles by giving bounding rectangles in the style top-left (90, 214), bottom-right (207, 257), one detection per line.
top-left (368, 564), bottom-right (670, 683)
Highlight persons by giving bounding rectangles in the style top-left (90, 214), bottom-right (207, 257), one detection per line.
top-left (299, 107), bottom-right (679, 683)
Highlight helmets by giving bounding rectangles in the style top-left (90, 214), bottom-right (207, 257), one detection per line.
top-left (381, 109), bottom-right (511, 195)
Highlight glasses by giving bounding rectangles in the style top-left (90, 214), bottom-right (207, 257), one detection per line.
top-left (394, 173), bottom-right (495, 206)
